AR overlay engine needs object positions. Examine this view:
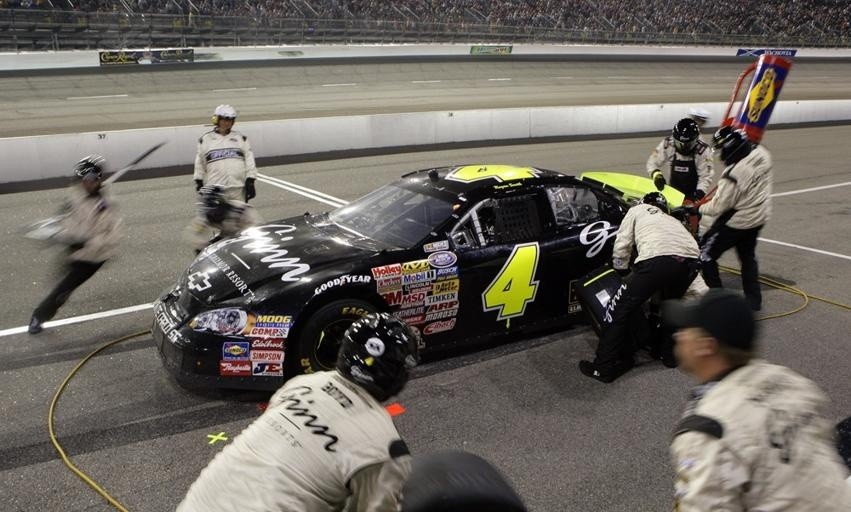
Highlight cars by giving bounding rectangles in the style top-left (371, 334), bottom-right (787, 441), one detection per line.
top-left (152, 165), bottom-right (703, 396)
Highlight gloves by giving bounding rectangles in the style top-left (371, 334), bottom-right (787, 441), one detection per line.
top-left (245, 176), bottom-right (256, 203)
top-left (653, 171), bottom-right (666, 190)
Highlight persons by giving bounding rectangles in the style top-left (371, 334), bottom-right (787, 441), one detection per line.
top-left (389, 451), bottom-right (537, 510)
top-left (190, 102), bottom-right (258, 258)
top-left (0, 0), bottom-right (851, 48)
top-left (22, 152), bottom-right (125, 335)
top-left (186, 185), bottom-right (267, 255)
top-left (656, 290), bottom-right (851, 512)
top-left (167, 311), bottom-right (415, 512)
top-left (647, 118), bottom-right (717, 219)
top-left (684, 126), bottom-right (775, 310)
top-left (577, 189), bottom-right (705, 383)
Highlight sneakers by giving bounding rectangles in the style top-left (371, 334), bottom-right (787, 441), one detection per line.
top-left (579, 359), bottom-right (613, 386)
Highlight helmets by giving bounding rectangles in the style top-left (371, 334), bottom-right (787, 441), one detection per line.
top-left (639, 192), bottom-right (670, 214)
top-left (714, 126), bottom-right (745, 150)
top-left (671, 117), bottom-right (701, 142)
top-left (213, 103), bottom-right (236, 125)
top-left (337, 312), bottom-right (421, 399)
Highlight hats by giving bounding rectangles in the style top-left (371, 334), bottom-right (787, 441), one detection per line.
top-left (664, 286), bottom-right (754, 339)
